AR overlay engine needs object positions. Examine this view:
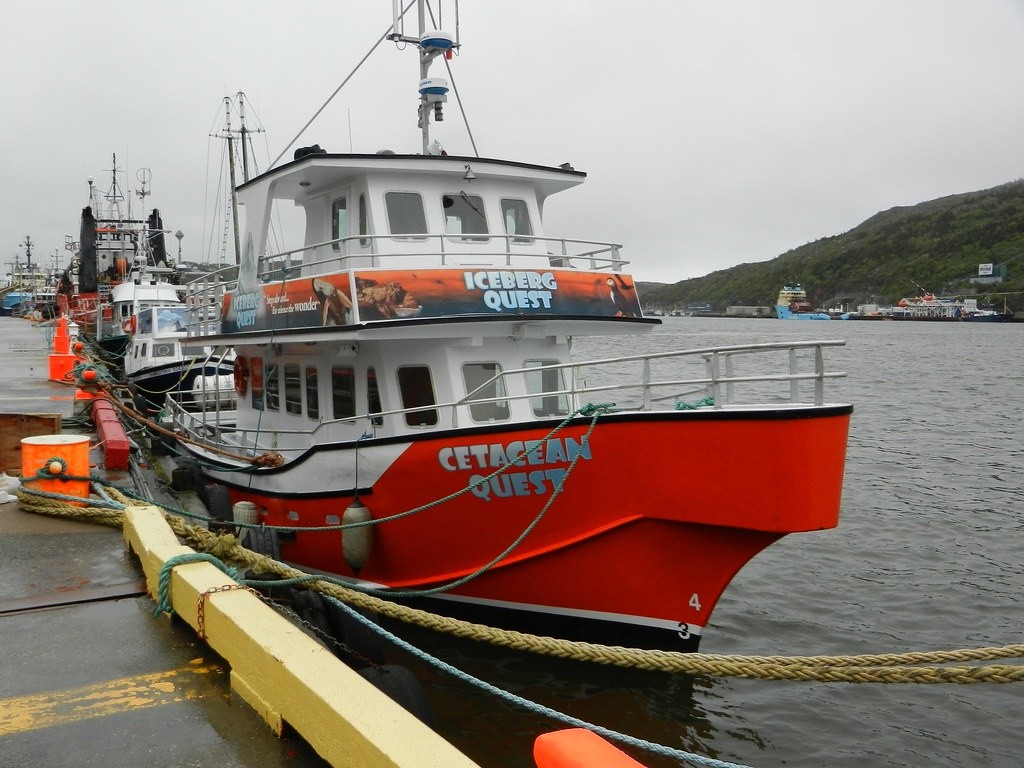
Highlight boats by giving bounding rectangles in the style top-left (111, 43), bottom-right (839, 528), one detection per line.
top-left (150, 0), bottom-right (855, 664)
top-left (774, 280), bottom-right (898, 320)
top-left (881, 279), bottom-right (1015, 321)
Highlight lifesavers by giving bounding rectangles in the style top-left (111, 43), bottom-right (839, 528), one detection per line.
top-left (131, 315), bottom-right (136, 330)
top-left (122, 320), bottom-right (135, 332)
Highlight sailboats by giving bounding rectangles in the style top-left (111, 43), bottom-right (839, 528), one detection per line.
top-left (57, 151), bottom-right (243, 407)
top-left (0, 233), bottom-right (61, 317)
